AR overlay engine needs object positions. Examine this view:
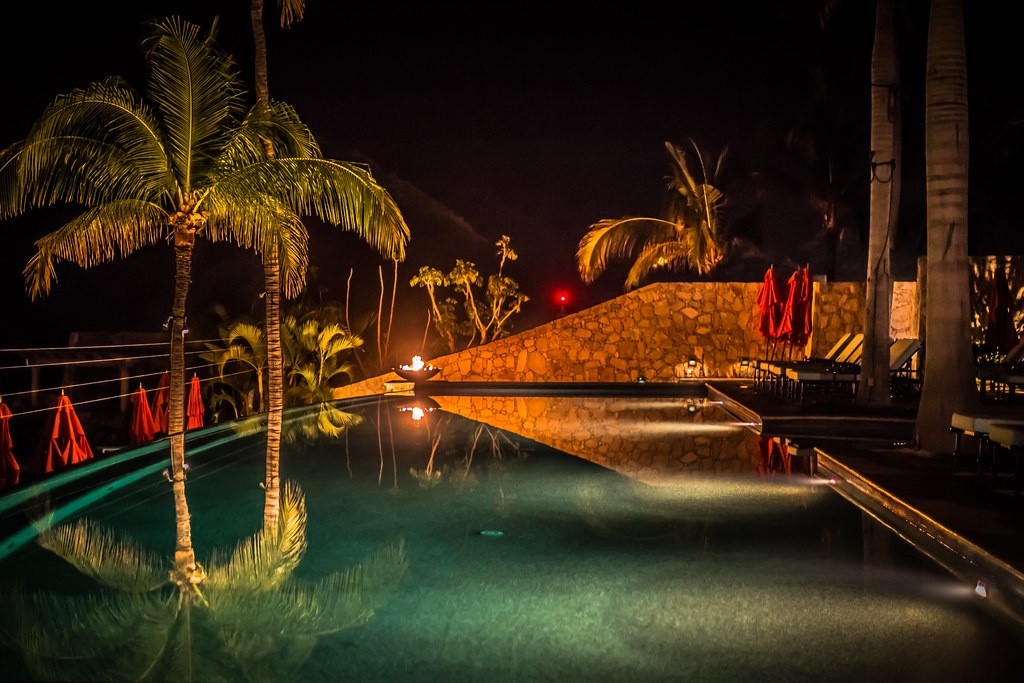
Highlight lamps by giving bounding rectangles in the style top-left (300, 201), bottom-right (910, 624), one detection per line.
top-left (395, 397), bottom-right (441, 421)
top-left (390, 355), bottom-right (441, 383)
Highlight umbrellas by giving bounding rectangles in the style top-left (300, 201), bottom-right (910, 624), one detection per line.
top-left (46, 388), bottom-right (95, 473)
top-left (755, 437), bottom-right (792, 480)
top-left (757, 263), bottom-right (812, 361)
top-left (129, 370), bottom-right (205, 443)
top-left (0, 396), bottom-right (20, 484)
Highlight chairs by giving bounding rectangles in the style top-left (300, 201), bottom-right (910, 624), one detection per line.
top-left (754, 332), bottom-right (919, 409)
top-left (977, 341), bottom-right (1024, 401)
top-left (950, 410), bottom-right (1024, 475)
